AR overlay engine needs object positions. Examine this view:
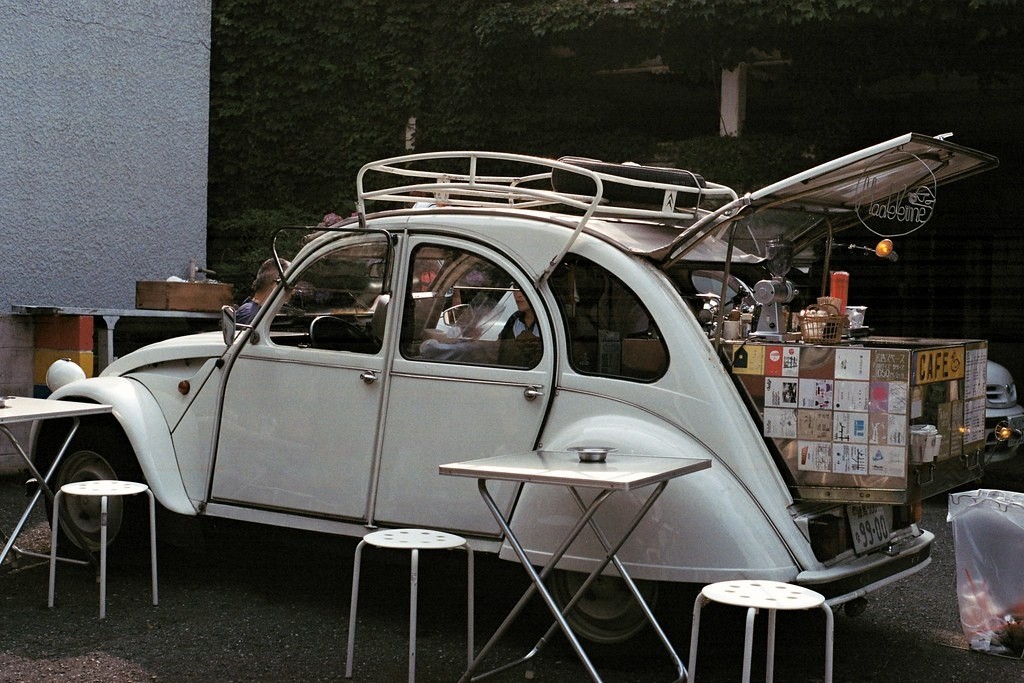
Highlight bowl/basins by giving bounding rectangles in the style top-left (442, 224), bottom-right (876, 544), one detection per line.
top-left (0, 396), bottom-right (15, 408)
top-left (567, 447), bottom-right (618, 460)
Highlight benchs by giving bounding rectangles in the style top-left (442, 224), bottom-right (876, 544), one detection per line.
top-left (499, 332), bottom-right (667, 383)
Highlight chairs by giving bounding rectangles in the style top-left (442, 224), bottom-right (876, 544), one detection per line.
top-left (369, 291), bottom-right (431, 354)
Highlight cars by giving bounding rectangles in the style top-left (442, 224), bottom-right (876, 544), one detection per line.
top-left (29, 133), bottom-right (1003, 664)
top-left (985, 360), bottom-right (1023, 466)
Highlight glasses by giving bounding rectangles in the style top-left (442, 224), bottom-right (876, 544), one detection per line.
top-left (513, 282), bottom-right (518, 288)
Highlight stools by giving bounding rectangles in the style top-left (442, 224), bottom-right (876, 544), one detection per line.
top-left (344, 528), bottom-right (475, 683)
top-left (47, 479), bottom-right (160, 622)
top-left (686, 578), bottom-right (836, 682)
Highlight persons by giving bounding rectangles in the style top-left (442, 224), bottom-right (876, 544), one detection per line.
top-left (235, 258), bottom-right (297, 331)
top-left (499, 282), bottom-right (541, 367)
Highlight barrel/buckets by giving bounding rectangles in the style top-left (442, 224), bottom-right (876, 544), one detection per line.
top-left (909, 424), bottom-right (937, 462)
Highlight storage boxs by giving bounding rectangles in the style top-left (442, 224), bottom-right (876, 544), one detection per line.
top-left (134, 277), bottom-right (236, 312)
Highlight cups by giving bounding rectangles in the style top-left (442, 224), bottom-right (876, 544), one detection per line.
top-left (846, 307), bottom-right (866, 327)
top-left (985, 617), bottom-right (1007, 646)
top-left (962, 581), bottom-right (997, 619)
top-left (723, 321), bottom-right (739, 339)
top-left (830, 272), bottom-right (848, 315)
top-left (961, 606), bottom-right (987, 641)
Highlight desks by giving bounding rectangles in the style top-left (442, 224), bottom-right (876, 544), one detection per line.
top-left (437, 449), bottom-right (714, 683)
top-left (10, 305), bottom-right (226, 399)
top-left (0, 395), bottom-right (114, 593)
